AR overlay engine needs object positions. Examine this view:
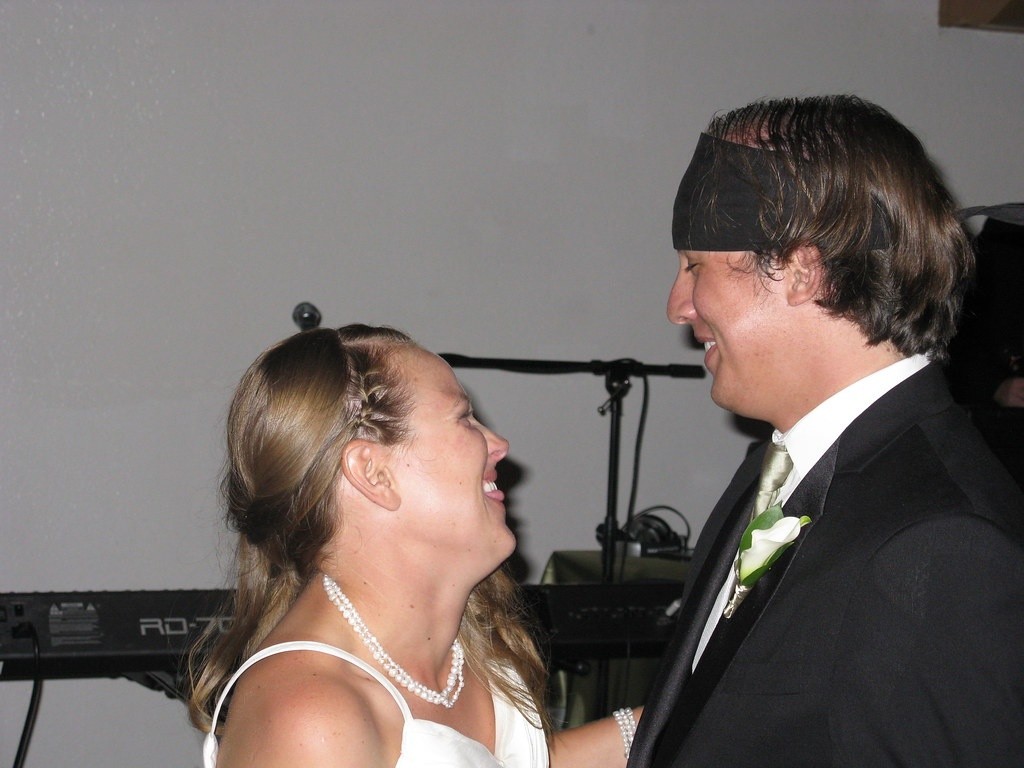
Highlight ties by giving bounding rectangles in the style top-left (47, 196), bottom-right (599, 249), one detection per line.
top-left (752, 441), bottom-right (793, 521)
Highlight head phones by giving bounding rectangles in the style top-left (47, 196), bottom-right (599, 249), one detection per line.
top-left (597, 515), bottom-right (682, 560)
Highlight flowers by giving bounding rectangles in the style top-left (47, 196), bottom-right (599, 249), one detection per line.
top-left (723, 498), bottom-right (813, 619)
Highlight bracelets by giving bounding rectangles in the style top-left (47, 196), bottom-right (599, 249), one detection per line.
top-left (613, 707), bottom-right (637, 758)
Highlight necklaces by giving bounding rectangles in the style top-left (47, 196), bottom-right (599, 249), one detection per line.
top-left (322, 571), bottom-right (465, 709)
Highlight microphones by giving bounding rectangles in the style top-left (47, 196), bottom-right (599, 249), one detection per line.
top-left (294, 302), bottom-right (321, 332)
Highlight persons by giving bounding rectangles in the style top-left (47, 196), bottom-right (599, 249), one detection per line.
top-left (629, 95), bottom-right (1024, 766)
top-left (936, 199), bottom-right (1023, 493)
top-left (187, 322), bottom-right (645, 768)
